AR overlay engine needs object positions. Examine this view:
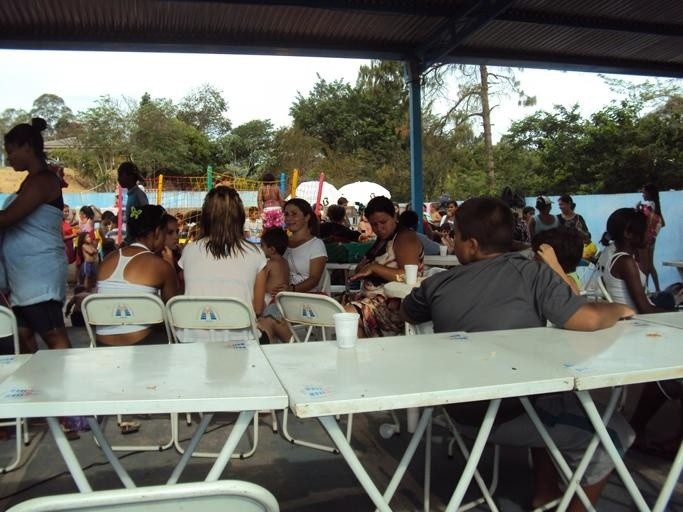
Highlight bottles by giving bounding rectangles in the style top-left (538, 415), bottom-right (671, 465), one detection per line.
top-left (251, 228), bottom-right (256, 241)
top-left (244, 230), bottom-right (249, 239)
top-left (358, 230), bottom-right (369, 242)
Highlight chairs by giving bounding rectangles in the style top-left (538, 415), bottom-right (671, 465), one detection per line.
top-left (290, 255), bottom-right (461, 329)
top-left (165, 295), bottom-right (278, 459)
top-left (81, 293), bottom-right (191, 451)
top-left (576, 242), bottom-right (612, 303)
top-left (3, 478), bottom-right (281, 512)
top-left (0, 304), bottom-right (30, 474)
top-left (275, 291), bottom-right (401, 456)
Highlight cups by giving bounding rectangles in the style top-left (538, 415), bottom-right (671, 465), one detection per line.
top-left (331, 313), bottom-right (360, 349)
top-left (404, 263), bottom-right (417, 285)
top-left (439, 246), bottom-right (447, 256)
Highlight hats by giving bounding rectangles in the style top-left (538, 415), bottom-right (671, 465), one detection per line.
top-left (536, 196), bottom-right (554, 206)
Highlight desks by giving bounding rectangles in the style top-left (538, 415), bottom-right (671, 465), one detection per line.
top-left (0, 340), bottom-right (289, 493)
top-left (0, 353), bottom-right (33, 383)
top-left (261, 330), bottom-right (575, 512)
top-left (661, 261), bottom-right (683, 281)
top-left (468, 318), bottom-right (683, 511)
top-left (631, 309), bottom-right (682, 329)
